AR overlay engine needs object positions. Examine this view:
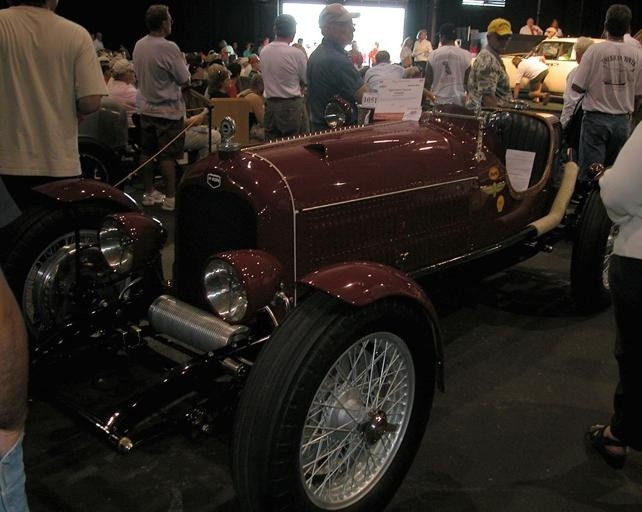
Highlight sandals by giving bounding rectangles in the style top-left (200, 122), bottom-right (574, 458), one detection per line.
top-left (533, 98), bottom-right (540, 103)
top-left (543, 93), bottom-right (550, 105)
top-left (589, 424), bottom-right (629, 470)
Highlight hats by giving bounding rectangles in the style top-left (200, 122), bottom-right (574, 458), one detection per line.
top-left (248, 54), bottom-right (260, 64)
top-left (119, 48), bottom-right (127, 53)
top-left (319, 3), bottom-right (360, 29)
top-left (437, 23), bottom-right (458, 34)
top-left (112, 51), bottom-right (119, 57)
top-left (488, 18), bottom-right (512, 36)
top-left (98, 57), bottom-right (110, 66)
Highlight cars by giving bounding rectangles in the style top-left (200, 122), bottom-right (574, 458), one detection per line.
top-left (0, 100), bottom-right (619, 512)
top-left (472, 38), bottom-right (607, 99)
top-left (78, 89), bottom-right (215, 192)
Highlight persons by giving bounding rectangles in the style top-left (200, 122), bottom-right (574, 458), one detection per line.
top-left (400, 37), bottom-right (413, 68)
top-left (573, 5), bottom-right (642, 185)
top-left (519, 18), bottom-right (543, 35)
top-left (412, 29), bottom-right (433, 68)
top-left (104, 59), bottom-right (138, 130)
top-left (184, 107), bottom-right (221, 160)
top-left (561, 36), bottom-right (595, 127)
top-left (307, 3), bottom-right (369, 133)
top-left (551, 19), bottom-right (563, 37)
top-left (365, 51), bottom-right (420, 90)
top-left (347, 41), bottom-right (363, 69)
top-left (105, 57), bottom-right (122, 85)
top-left (134, 6), bottom-right (191, 210)
top-left (1, 1), bottom-right (109, 179)
top-left (1, 268), bottom-right (28, 511)
top-left (587, 121), bottom-right (642, 468)
top-left (94, 32), bottom-right (133, 62)
top-left (511, 55), bottom-right (550, 104)
top-left (463, 19), bottom-right (513, 109)
top-left (545, 28), bottom-right (558, 39)
top-left (193, 39), bottom-right (268, 99)
top-left (424, 23), bottom-right (472, 106)
top-left (292, 38), bottom-right (306, 55)
top-left (259, 15), bottom-right (309, 140)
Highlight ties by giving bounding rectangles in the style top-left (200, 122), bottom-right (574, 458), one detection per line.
top-left (530, 27), bottom-right (534, 35)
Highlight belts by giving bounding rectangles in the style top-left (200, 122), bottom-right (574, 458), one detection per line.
top-left (583, 111), bottom-right (633, 117)
top-left (267, 97), bottom-right (299, 100)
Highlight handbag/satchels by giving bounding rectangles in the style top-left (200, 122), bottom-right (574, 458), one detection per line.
top-left (603, 224), bottom-right (619, 290)
top-left (564, 95), bottom-right (584, 152)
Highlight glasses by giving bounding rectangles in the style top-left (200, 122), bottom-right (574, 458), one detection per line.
top-left (495, 34), bottom-right (513, 41)
top-left (338, 23), bottom-right (355, 29)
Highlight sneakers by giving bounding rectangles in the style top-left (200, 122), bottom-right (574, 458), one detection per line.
top-left (142, 190), bottom-right (166, 206)
top-left (161, 197), bottom-right (175, 211)
